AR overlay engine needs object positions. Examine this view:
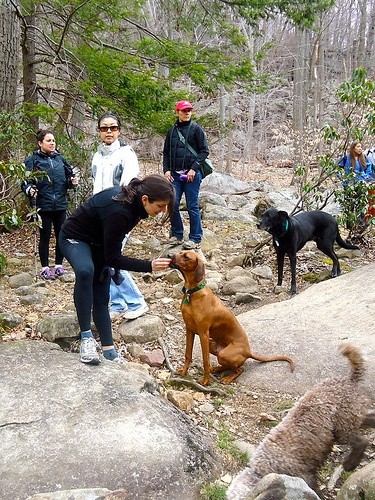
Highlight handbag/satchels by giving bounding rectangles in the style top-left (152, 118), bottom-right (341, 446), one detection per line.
top-left (199, 157), bottom-right (214, 178)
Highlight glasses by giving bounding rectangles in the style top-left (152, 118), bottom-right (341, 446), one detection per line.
top-left (99, 125), bottom-right (120, 132)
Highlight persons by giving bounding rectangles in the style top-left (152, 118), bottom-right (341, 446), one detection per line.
top-left (59, 174), bottom-right (177, 366)
top-left (90, 114), bottom-right (148, 319)
top-left (335, 141), bottom-right (375, 243)
top-left (20, 129), bottom-right (78, 280)
top-left (163, 100), bottom-right (209, 249)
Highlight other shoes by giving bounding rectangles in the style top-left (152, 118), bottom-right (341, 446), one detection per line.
top-left (109, 310), bottom-right (127, 322)
top-left (122, 305), bottom-right (149, 320)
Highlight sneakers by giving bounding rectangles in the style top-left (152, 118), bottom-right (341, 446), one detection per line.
top-left (182, 240), bottom-right (201, 250)
top-left (79, 337), bottom-right (102, 364)
top-left (103, 346), bottom-right (128, 365)
top-left (167, 236), bottom-right (184, 245)
top-left (55, 266), bottom-right (68, 276)
top-left (40, 268), bottom-right (56, 281)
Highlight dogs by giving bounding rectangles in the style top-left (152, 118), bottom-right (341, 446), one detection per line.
top-left (226, 340), bottom-right (365, 500)
top-left (168, 251), bottom-right (296, 388)
top-left (257, 208), bottom-right (361, 294)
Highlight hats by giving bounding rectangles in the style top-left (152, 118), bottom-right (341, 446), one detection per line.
top-left (175, 100), bottom-right (193, 111)
